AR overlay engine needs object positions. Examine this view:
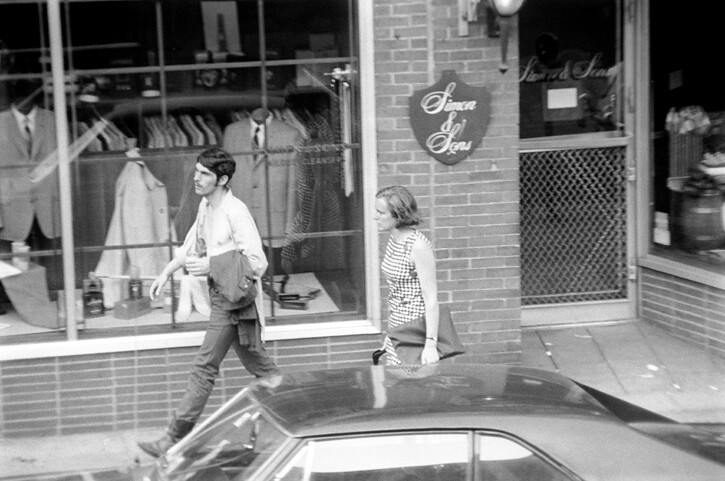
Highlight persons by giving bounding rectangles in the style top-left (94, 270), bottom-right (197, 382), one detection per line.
top-left (138, 148), bottom-right (284, 456)
top-left (372, 185), bottom-right (442, 366)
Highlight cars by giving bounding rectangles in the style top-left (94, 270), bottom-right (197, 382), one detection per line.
top-left (0, 356), bottom-right (725, 481)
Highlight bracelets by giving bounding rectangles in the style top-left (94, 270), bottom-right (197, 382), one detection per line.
top-left (424, 337), bottom-right (438, 341)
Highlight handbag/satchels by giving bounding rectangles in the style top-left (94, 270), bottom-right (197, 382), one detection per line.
top-left (387, 306), bottom-right (464, 365)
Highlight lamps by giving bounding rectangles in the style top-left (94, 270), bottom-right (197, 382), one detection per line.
top-left (486, 0), bottom-right (525, 73)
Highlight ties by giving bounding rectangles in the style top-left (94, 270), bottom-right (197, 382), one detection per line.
top-left (252, 128), bottom-right (260, 160)
top-left (23, 119), bottom-right (33, 157)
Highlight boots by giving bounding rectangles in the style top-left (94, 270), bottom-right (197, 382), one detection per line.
top-left (138, 419), bottom-right (193, 456)
